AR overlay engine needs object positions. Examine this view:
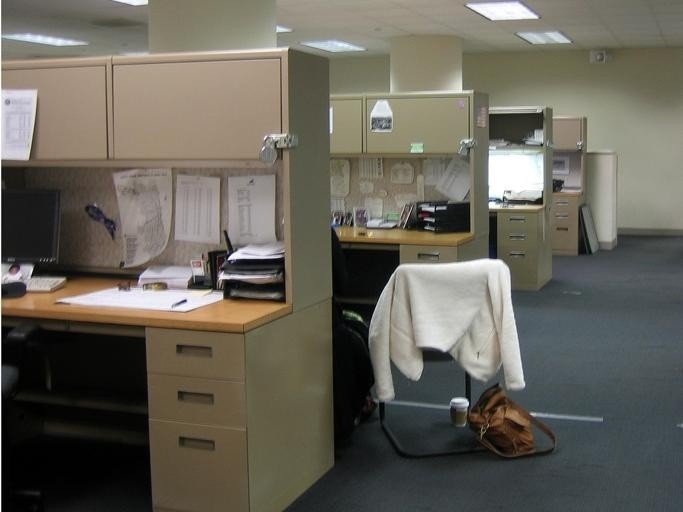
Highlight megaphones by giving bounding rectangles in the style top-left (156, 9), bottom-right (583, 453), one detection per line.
top-left (588, 50), bottom-right (609, 65)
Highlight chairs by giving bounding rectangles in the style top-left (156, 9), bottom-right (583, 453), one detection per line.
top-left (331, 224), bottom-right (496, 459)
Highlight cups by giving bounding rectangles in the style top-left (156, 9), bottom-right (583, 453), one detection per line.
top-left (450, 397), bottom-right (470, 427)
top-left (354, 207), bottom-right (367, 237)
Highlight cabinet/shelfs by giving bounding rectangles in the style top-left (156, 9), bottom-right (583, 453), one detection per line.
top-left (331, 90), bottom-right (488, 333)
top-left (488, 105), bottom-right (554, 293)
top-left (551, 116), bottom-right (587, 256)
top-left (1, 46), bottom-right (337, 511)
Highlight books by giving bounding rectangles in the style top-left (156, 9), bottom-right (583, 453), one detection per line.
top-left (234, 259), bottom-right (283, 272)
top-left (214, 254), bottom-right (227, 282)
top-left (205, 250), bottom-right (228, 288)
top-left (523, 138), bottom-right (542, 146)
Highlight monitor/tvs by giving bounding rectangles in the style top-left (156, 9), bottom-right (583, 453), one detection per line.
top-left (1, 188), bottom-right (63, 265)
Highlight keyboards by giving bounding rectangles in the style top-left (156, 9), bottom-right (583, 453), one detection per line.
top-left (25, 276), bottom-right (67, 293)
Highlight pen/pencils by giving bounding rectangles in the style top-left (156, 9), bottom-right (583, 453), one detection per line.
top-left (171, 299), bottom-right (187, 307)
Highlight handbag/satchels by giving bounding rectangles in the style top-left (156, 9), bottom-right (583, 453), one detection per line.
top-left (464, 382), bottom-right (558, 460)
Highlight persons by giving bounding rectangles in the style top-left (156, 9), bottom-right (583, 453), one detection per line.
top-left (0, 264), bottom-right (24, 284)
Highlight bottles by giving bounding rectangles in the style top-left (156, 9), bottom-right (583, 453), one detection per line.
top-left (503, 189), bottom-right (512, 207)
